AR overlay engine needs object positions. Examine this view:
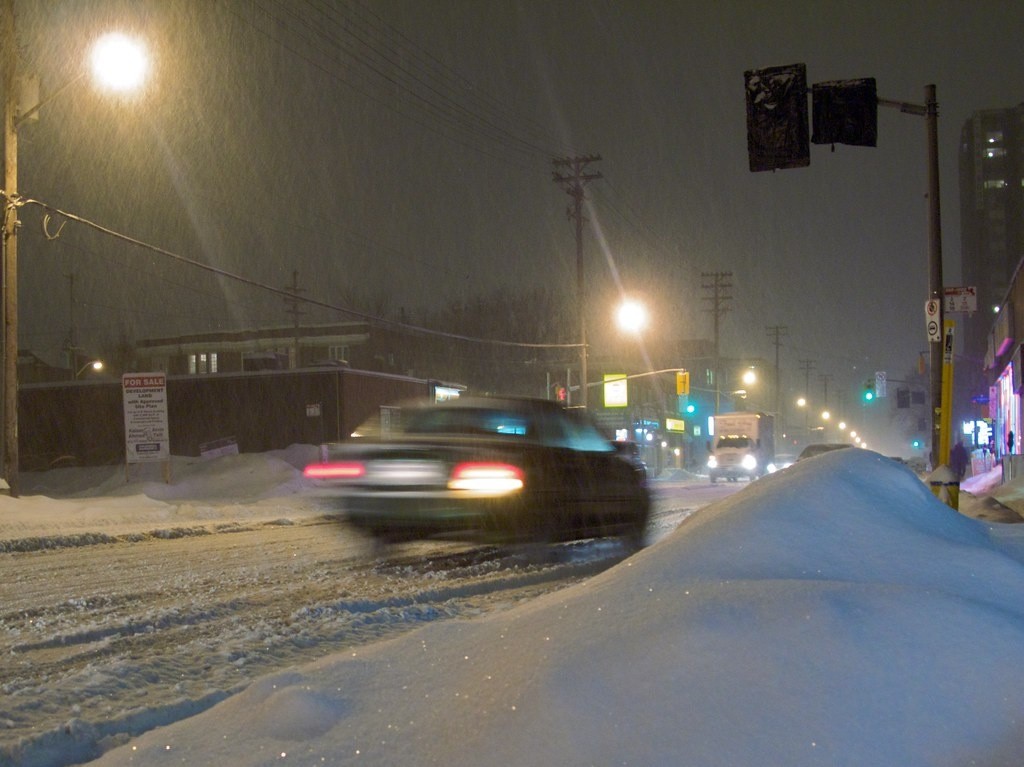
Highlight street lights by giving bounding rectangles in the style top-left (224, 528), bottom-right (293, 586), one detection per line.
top-left (0, 30), bottom-right (159, 496)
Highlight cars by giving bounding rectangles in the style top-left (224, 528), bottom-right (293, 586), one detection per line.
top-left (302, 395), bottom-right (652, 547)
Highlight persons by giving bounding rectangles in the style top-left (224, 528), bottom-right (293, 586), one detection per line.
top-left (953, 441), bottom-right (969, 480)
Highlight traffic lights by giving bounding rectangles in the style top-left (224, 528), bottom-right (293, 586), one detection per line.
top-left (865, 383), bottom-right (874, 402)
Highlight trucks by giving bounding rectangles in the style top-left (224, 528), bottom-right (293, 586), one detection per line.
top-left (706, 412), bottom-right (775, 484)
